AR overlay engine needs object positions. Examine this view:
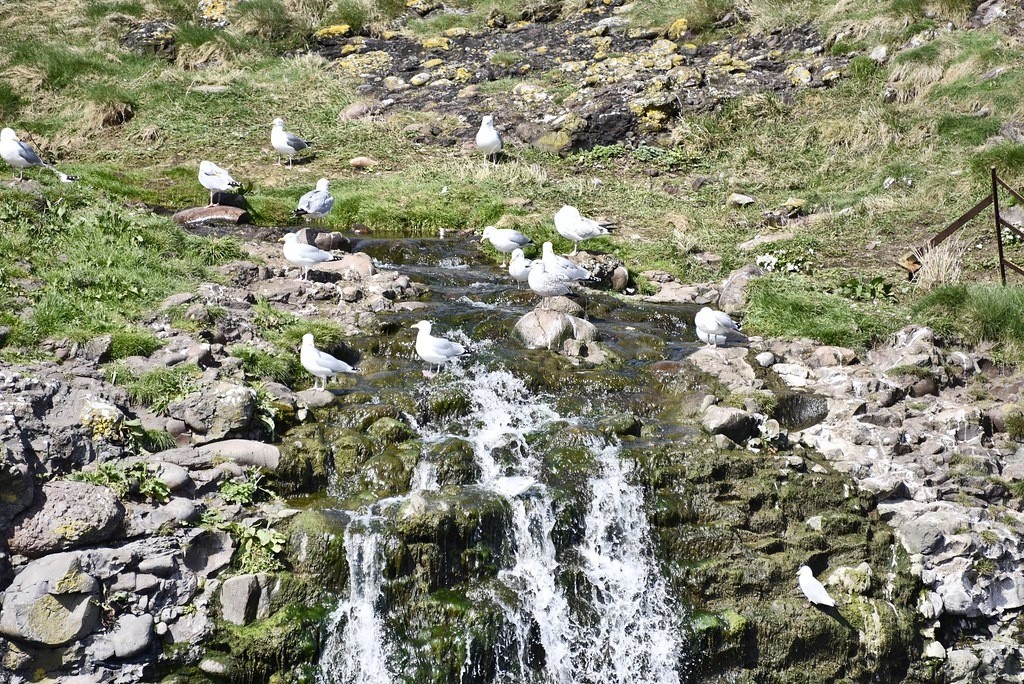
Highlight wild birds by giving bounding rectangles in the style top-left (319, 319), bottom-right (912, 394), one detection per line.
top-left (198, 160), bottom-right (242, 209)
top-left (277, 232), bottom-right (343, 283)
top-left (552, 204), bottom-right (612, 256)
top-left (0, 127), bottom-right (47, 182)
top-left (796, 566), bottom-right (836, 607)
top-left (410, 320), bottom-right (466, 374)
top-left (270, 118), bottom-right (316, 171)
top-left (476, 114), bottom-right (504, 165)
top-left (694, 306), bottom-right (741, 351)
top-left (300, 333), bottom-right (359, 392)
top-left (294, 178), bottom-right (335, 228)
top-left (508, 241), bottom-right (592, 312)
top-left (480, 226), bottom-right (536, 268)
